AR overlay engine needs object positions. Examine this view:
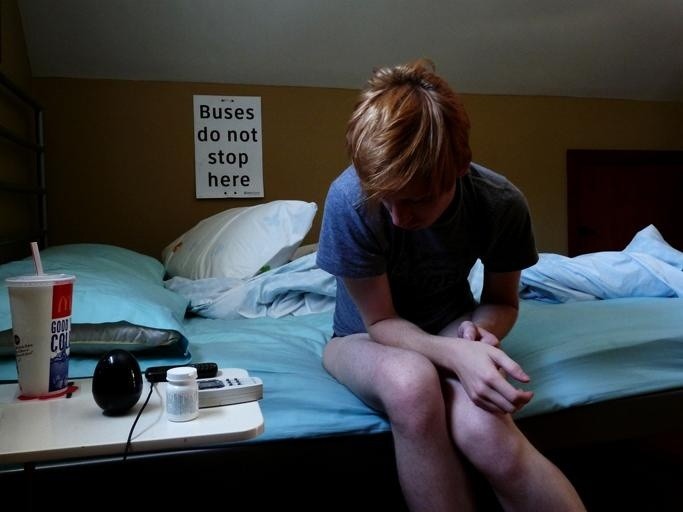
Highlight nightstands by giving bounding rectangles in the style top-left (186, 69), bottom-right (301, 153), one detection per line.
top-left (1, 368), bottom-right (265, 474)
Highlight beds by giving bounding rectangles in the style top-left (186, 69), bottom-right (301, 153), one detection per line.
top-left (0, 224), bottom-right (683, 512)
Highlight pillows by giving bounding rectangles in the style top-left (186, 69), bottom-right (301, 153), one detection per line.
top-left (163, 199), bottom-right (318, 289)
top-left (0, 244), bottom-right (194, 382)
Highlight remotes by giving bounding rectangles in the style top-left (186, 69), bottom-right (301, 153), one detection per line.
top-left (148, 361), bottom-right (219, 383)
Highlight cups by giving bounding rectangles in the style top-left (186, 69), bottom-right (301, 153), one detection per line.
top-left (5, 275), bottom-right (76, 396)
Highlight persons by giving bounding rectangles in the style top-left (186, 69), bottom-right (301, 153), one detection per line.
top-left (317, 65), bottom-right (586, 512)
